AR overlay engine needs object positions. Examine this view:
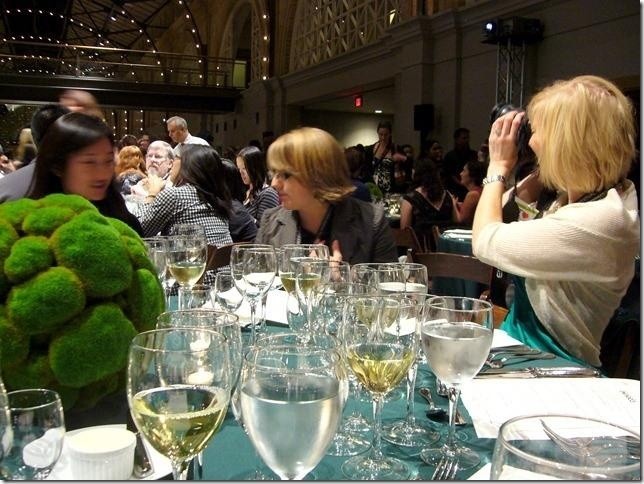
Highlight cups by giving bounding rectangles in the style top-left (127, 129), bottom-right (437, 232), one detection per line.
top-left (278, 242), bottom-right (329, 313)
top-left (306, 282), bottom-right (378, 456)
top-left (419, 295), bottom-right (493, 468)
top-left (349, 261), bottom-right (405, 330)
top-left (156, 310), bottom-right (242, 481)
top-left (376, 261), bottom-right (429, 338)
top-left (290, 255), bottom-right (348, 334)
top-left (126, 328), bottom-right (231, 481)
top-left (491, 413), bottom-right (643, 480)
top-left (178, 284), bottom-right (214, 311)
top-left (0, 388), bottom-right (64, 480)
top-left (139, 237), bottom-right (167, 284)
top-left (254, 331), bottom-right (344, 357)
top-left (340, 294), bottom-right (418, 481)
top-left (165, 234), bottom-right (207, 307)
top-left (167, 223), bottom-right (205, 261)
top-left (286, 289), bottom-right (318, 372)
top-left (238, 345), bottom-right (346, 482)
top-left (213, 270), bottom-right (245, 314)
top-left (229, 243), bottom-right (278, 344)
top-left (368, 289), bottom-right (449, 449)
top-left (246, 246), bottom-right (287, 339)
top-left (61, 425), bottom-right (136, 479)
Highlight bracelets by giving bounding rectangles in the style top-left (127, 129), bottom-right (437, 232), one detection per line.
top-left (482, 174), bottom-right (509, 188)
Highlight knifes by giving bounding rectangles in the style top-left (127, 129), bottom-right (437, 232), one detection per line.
top-left (476, 365), bottom-right (600, 377)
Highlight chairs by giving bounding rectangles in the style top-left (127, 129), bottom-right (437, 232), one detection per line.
top-left (392, 225), bottom-right (422, 265)
top-left (407, 246), bottom-right (497, 303)
top-left (431, 224), bottom-right (441, 253)
top-left (206, 240), bottom-right (256, 273)
top-left (600, 318), bottom-right (639, 380)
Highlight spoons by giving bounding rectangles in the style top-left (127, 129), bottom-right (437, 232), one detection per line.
top-left (418, 387), bottom-right (449, 424)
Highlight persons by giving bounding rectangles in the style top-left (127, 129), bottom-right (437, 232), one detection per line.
top-left (399, 146), bottom-right (414, 162)
top-left (369, 119), bottom-right (406, 191)
top-left (346, 146), bottom-right (371, 179)
top-left (25, 112), bottom-right (146, 236)
top-left (165, 116), bottom-right (208, 154)
top-left (237, 146), bottom-right (279, 229)
top-left (139, 147), bottom-right (233, 248)
top-left (222, 167), bottom-right (257, 242)
top-left (0, 105), bottom-right (69, 203)
top-left (445, 163), bottom-right (484, 226)
top-left (115, 147), bottom-right (142, 194)
top-left (400, 162), bottom-right (454, 254)
top-left (132, 141), bottom-right (176, 207)
top-left (252, 126), bottom-right (396, 276)
top-left (444, 128), bottom-right (477, 179)
top-left (414, 141), bottom-right (445, 178)
top-left (470, 75), bottom-right (642, 375)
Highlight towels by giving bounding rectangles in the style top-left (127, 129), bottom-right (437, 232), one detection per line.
top-left (31, 424), bottom-right (175, 480)
top-left (491, 327), bottom-right (523, 347)
top-left (202, 271), bottom-right (304, 326)
top-left (455, 379), bottom-right (641, 442)
top-left (469, 463), bottom-right (552, 480)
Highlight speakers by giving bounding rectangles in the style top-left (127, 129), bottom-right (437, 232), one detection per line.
top-left (413, 104), bottom-right (434, 132)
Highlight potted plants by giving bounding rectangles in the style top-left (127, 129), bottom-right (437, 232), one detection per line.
top-left (1, 193), bottom-right (167, 431)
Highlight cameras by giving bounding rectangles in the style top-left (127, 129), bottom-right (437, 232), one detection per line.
top-left (490, 102), bottom-right (535, 161)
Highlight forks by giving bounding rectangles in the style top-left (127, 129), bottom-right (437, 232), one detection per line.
top-left (557, 441), bottom-right (640, 466)
top-left (542, 429), bottom-right (640, 456)
top-left (430, 453), bottom-right (459, 479)
top-left (539, 416), bottom-right (643, 449)
top-left (483, 352), bottom-right (555, 369)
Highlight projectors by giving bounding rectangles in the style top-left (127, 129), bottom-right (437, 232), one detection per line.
top-left (479, 15), bottom-right (544, 46)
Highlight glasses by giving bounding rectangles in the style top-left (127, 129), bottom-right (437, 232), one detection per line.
top-left (269, 167), bottom-right (294, 181)
top-left (148, 153), bottom-right (168, 157)
top-left (63, 153), bottom-right (118, 170)
